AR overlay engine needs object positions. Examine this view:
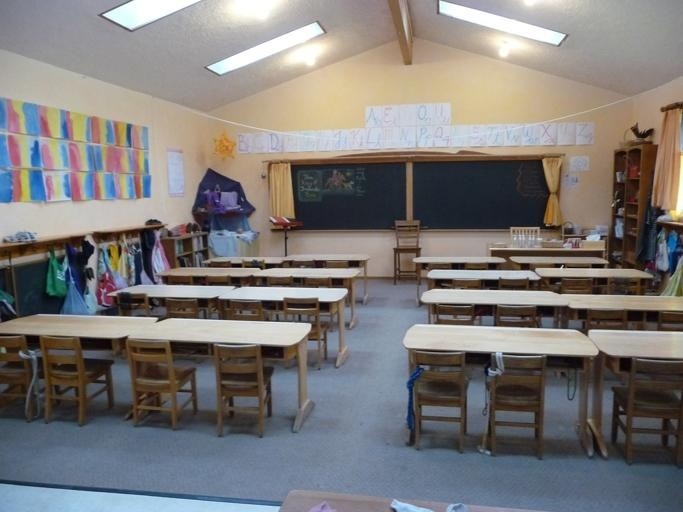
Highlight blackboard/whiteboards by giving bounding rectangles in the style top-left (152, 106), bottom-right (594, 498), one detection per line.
top-left (267, 159), bottom-right (562, 232)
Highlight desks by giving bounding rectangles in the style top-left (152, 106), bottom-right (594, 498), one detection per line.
top-left (282, 253), bottom-right (372, 304)
top-left (0, 314), bottom-right (158, 420)
top-left (202, 255), bottom-right (285, 271)
top-left (128, 315), bottom-right (317, 434)
top-left (588, 330), bottom-right (683, 461)
top-left (419, 288), bottom-right (571, 326)
top-left (108, 284), bottom-right (236, 318)
top-left (203, 256), bottom-right (286, 267)
top-left (509, 256), bottom-right (608, 268)
top-left (428, 269), bottom-right (542, 290)
top-left (412, 256), bottom-right (506, 285)
top-left (536, 268), bottom-right (654, 294)
top-left (154, 265), bottom-right (260, 286)
top-left (561, 295), bottom-right (683, 382)
top-left (488, 243), bottom-right (606, 258)
top-left (402, 323), bottom-right (600, 459)
top-left (251, 266), bottom-right (362, 330)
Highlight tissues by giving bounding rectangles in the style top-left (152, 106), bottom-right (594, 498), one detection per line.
top-left (580, 234), bottom-right (605, 249)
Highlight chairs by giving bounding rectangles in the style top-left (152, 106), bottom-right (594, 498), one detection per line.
top-left (452, 278), bottom-right (482, 290)
top-left (284, 296), bottom-right (330, 370)
top-left (168, 276), bottom-right (194, 285)
top-left (126, 339), bottom-right (198, 428)
top-left (498, 277), bottom-right (529, 290)
top-left (493, 303), bottom-right (539, 327)
top-left (211, 260), bottom-right (232, 267)
top-left (206, 275), bottom-right (231, 286)
top-left (166, 297), bottom-right (200, 318)
top-left (657, 310), bottom-right (683, 332)
top-left (213, 344), bottom-right (274, 439)
top-left (266, 276), bottom-right (294, 286)
top-left (242, 259), bottom-right (264, 268)
top-left (305, 276), bottom-right (333, 287)
top-left (0, 335), bottom-right (34, 421)
top-left (218, 301), bottom-right (266, 321)
top-left (465, 261), bottom-right (490, 268)
top-left (510, 227), bottom-right (540, 245)
top-left (116, 292), bottom-right (152, 317)
top-left (428, 303), bottom-right (477, 325)
top-left (39, 334), bottom-right (115, 426)
top-left (313, 260), bottom-right (328, 268)
top-left (394, 220), bottom-right (422, 285)
top-left (565, 262), bottom-right (591, 268)
top-left (601, 278), bottom-right (645, 295)
top-left (586, 308), bottom-right (627, 329)
top-left (410, 349), bottom-right (469, 455)
top-left (482, 354), bottom-right (546, 460)
top-left (611, 357), bottom-right (683, 469)
top-left (430, 261), bottom-right (452, 269)
top-left (529, 262), bottom-right (555, 268)
top-left (281, 259), bottom-right (298, 268)
top-left (560, 278), bottom-right (594, 295)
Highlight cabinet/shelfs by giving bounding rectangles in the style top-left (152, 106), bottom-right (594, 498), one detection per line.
top-left (0, 221), bottom-right (168, 324)
top-left (610, 144), bottom-right (659, 274)
top-left (159, 230), bottom-right (210, 269)
top-left (657, 219), bottom-right (683, 296)
